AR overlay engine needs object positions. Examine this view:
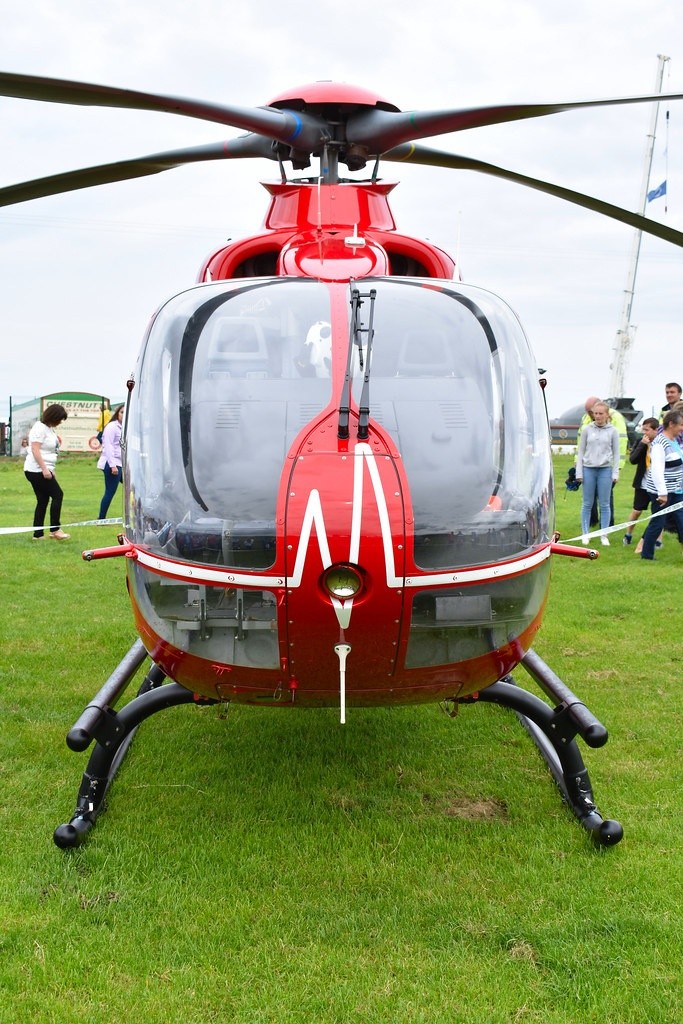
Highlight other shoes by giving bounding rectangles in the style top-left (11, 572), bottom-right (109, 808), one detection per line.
top-left (601, 537), bottom-right (610, 546)
top-left (623, 534), bottom-right (632, 544)
top-left (33, 536), bottom-right (45, 540)
top-left (654, 540), bottom-right (663, 549)
top-left (49, 528), bottom-right (69, 540)
top-left (582, 538), bottom-right (589, 545)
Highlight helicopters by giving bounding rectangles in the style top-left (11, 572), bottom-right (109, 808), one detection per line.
top-left (0, 66), bottom-right (682, 854)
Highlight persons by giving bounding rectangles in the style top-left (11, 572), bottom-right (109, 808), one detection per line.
top-left (96, 403), bottom-right (124, 526)
top-left (19, 405), bottom-right (71, 541)
top-left (623, 383), bottom-right (683, 560)
top-left (564, 396), bottom-right (628, 546)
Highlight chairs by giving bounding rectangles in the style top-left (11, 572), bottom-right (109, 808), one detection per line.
top-left (190, 317), bottom-right (494, 490)
